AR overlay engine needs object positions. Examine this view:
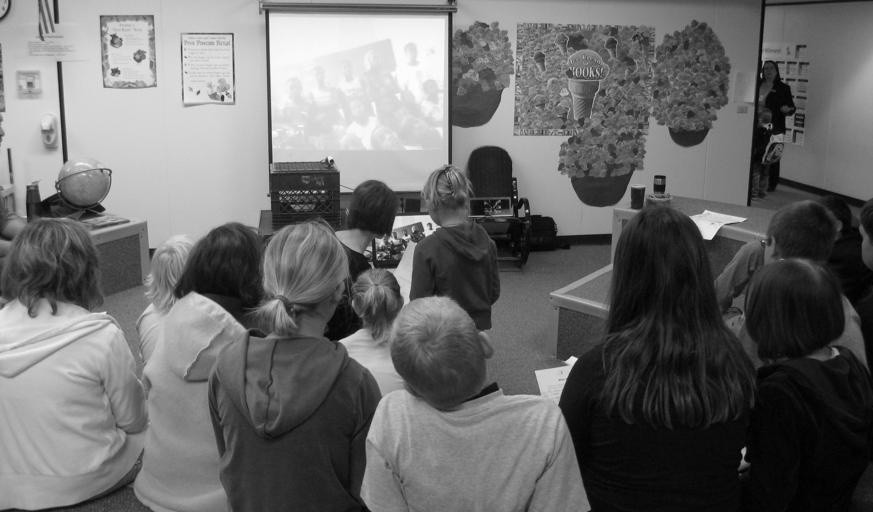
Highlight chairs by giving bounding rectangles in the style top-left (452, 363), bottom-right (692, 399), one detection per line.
top-left (460, 147), bottom-right (531, 267)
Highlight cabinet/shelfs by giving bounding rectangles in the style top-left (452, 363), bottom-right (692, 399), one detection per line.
top-left (548, 190), bottom-right (779, 368)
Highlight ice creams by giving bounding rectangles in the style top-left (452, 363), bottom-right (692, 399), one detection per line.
top-left (533, 51), bottom-right (546, 72)
top-left (565, 49), bottom-right (610, 121)
top-left (604, 37), bottom-right (617, 58)
top-left (554, 32), bottom-right (569, 55)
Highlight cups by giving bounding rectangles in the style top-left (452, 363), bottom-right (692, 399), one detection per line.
top-left (651, 176), bottom-right (668, 198)
top-left (628, 185), bottom-right (646, 210)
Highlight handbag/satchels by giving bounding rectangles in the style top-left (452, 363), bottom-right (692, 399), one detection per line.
top-left (519, 215), bottom-right (556, 251)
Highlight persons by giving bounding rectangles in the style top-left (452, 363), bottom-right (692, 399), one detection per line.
top-left (756, 61), bottom-right (796, 192)
top-left (751, 109), bottom-right (774, 198)
top-left (558, 193), bottom-right (873, 512)
top-left (132, 165), bottom-right (591, 512)
top-left (1, 216), bottom-right (148, 512)
top-left (270, 41), bottom-right (446, 160)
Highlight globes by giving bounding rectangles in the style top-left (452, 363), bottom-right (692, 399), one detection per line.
top-left (55, 156), bottom-right (112, 222)
